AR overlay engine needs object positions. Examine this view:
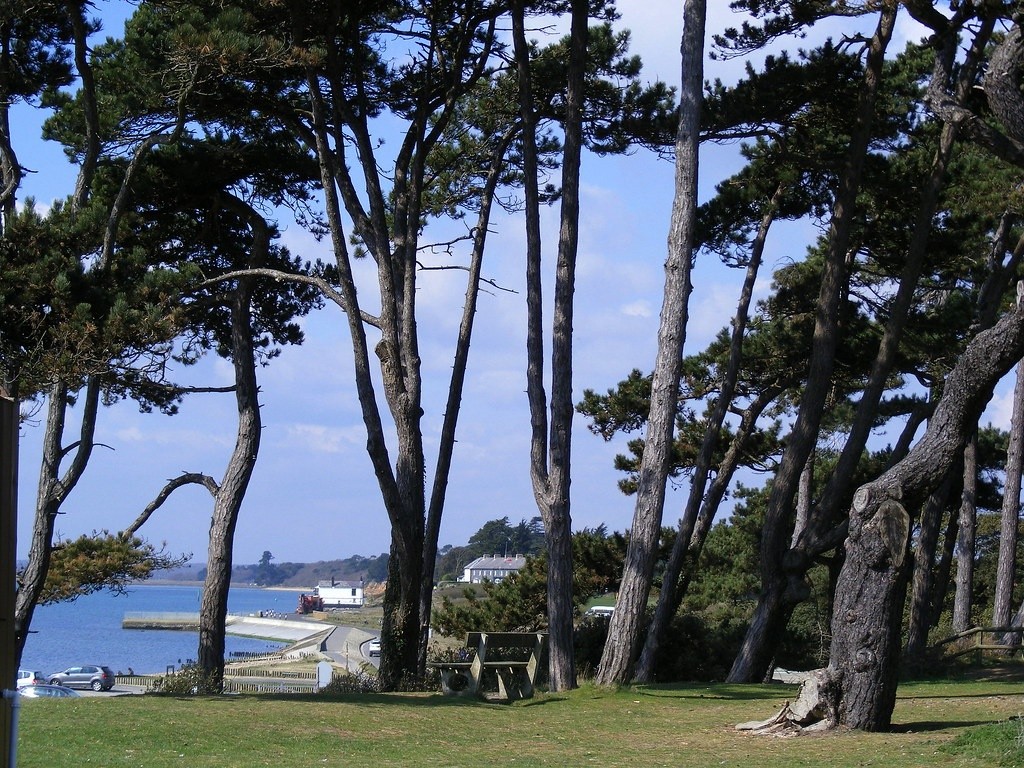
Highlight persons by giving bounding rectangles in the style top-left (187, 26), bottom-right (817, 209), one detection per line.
top-left (127, 668), bottom-right (133, 675)
top-left (117, 671), bottom-right (123, 676)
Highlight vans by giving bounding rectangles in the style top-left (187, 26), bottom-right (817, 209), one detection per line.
top-left (585, 606), bottom-right (615, 619)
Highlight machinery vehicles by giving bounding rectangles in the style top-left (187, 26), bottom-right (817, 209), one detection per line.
top-left (296, 593), bottom-right (325, 614)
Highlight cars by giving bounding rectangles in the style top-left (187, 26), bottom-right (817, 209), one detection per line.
top-left (15, 685), bottom-right (83, 698)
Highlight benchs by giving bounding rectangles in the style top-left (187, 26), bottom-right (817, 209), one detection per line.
top-left (430, 632), bottom-right (550, 701)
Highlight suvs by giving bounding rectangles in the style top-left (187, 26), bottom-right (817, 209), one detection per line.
top-left (16, 670), bottom-right (46, 690)
top-left (46, 665), bottom-right (116, 692)
top-left (368, 640), bottom-right (382, 657)
